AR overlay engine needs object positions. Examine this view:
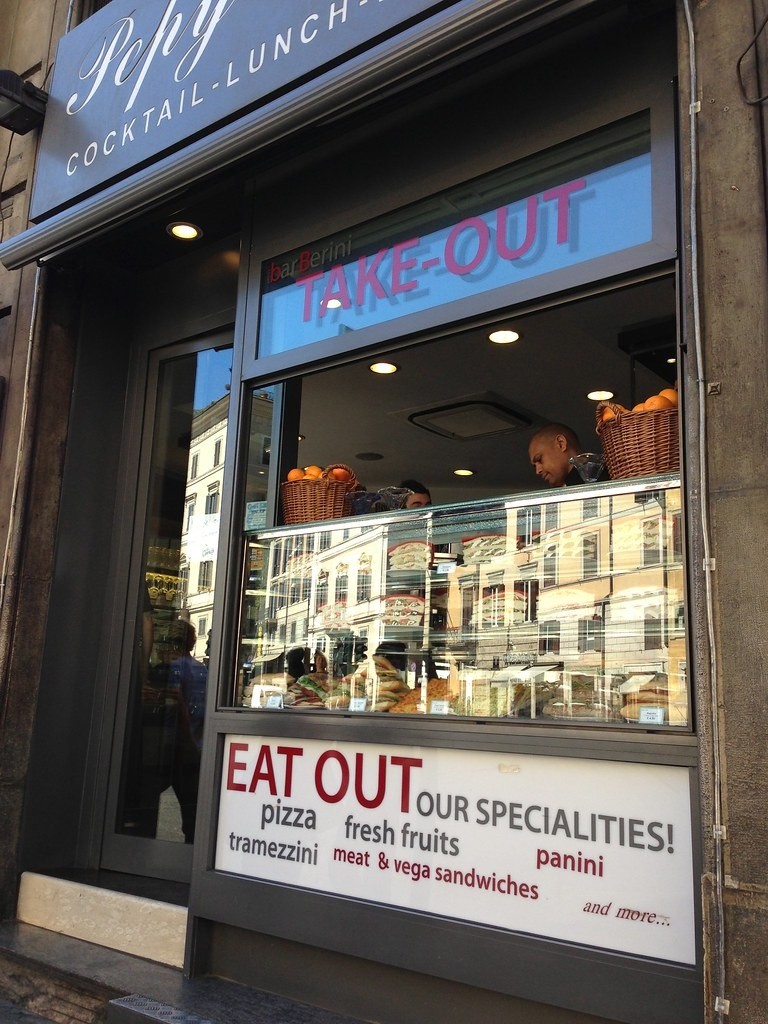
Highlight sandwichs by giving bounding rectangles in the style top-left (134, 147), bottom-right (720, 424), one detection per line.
top-left (621, 681), bottom-right (669, 722)
top-left (544, 680), bottom-right (624, 719)
top-left (286, 514), bottom-right (680, 621)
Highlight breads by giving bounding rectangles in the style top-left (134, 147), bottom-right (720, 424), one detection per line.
top-left (242, 670), bottom-right (296, 709)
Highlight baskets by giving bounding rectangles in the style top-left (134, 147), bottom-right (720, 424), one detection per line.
top-left (281, 463), bottom-right (361, 526)
top-left (595, 382), bottom-right (680, 480)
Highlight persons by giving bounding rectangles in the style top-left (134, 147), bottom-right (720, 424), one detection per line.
top-left (528, 423), bottom-right (609, 487)
top-left (398, 480), bottom-right (431, 509)
top-left (130, 619), bottom-right (205, 843)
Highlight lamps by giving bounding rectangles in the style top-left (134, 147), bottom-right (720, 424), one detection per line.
top-left (453, 467), bottom-right (474, 475)
top-left (587, 387), bottom-right (614, 400)
top-left (487, 325), bottom-right (520, 344)
top-left (166, 222), bottom-right (203, 241)
top-left (369, 358), bottom-right (398, 375)
top-left (387, 388), bottom-right (553, 442)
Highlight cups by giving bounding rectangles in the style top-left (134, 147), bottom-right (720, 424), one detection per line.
top-left (378, 487), bottom-right (415, 511)
top-left (347, 492), bottom-right (381, 515)
top-left (569, 453), bottom-right (606, 483)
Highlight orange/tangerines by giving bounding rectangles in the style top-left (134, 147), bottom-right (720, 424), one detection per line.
top-left (287, 465), bottom-right (352, 482)
top-left (600, 389), bottom-right (678, 421)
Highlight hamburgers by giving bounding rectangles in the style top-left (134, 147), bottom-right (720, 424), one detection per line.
top-left (296, 654), bottom-right (406, 713)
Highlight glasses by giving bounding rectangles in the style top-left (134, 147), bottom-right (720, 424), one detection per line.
top-left (157, 636), bottom-right (190, 646)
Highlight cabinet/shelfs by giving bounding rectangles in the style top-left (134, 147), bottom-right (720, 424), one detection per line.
top-left (192, 470), bottom-right (703, 1021)
